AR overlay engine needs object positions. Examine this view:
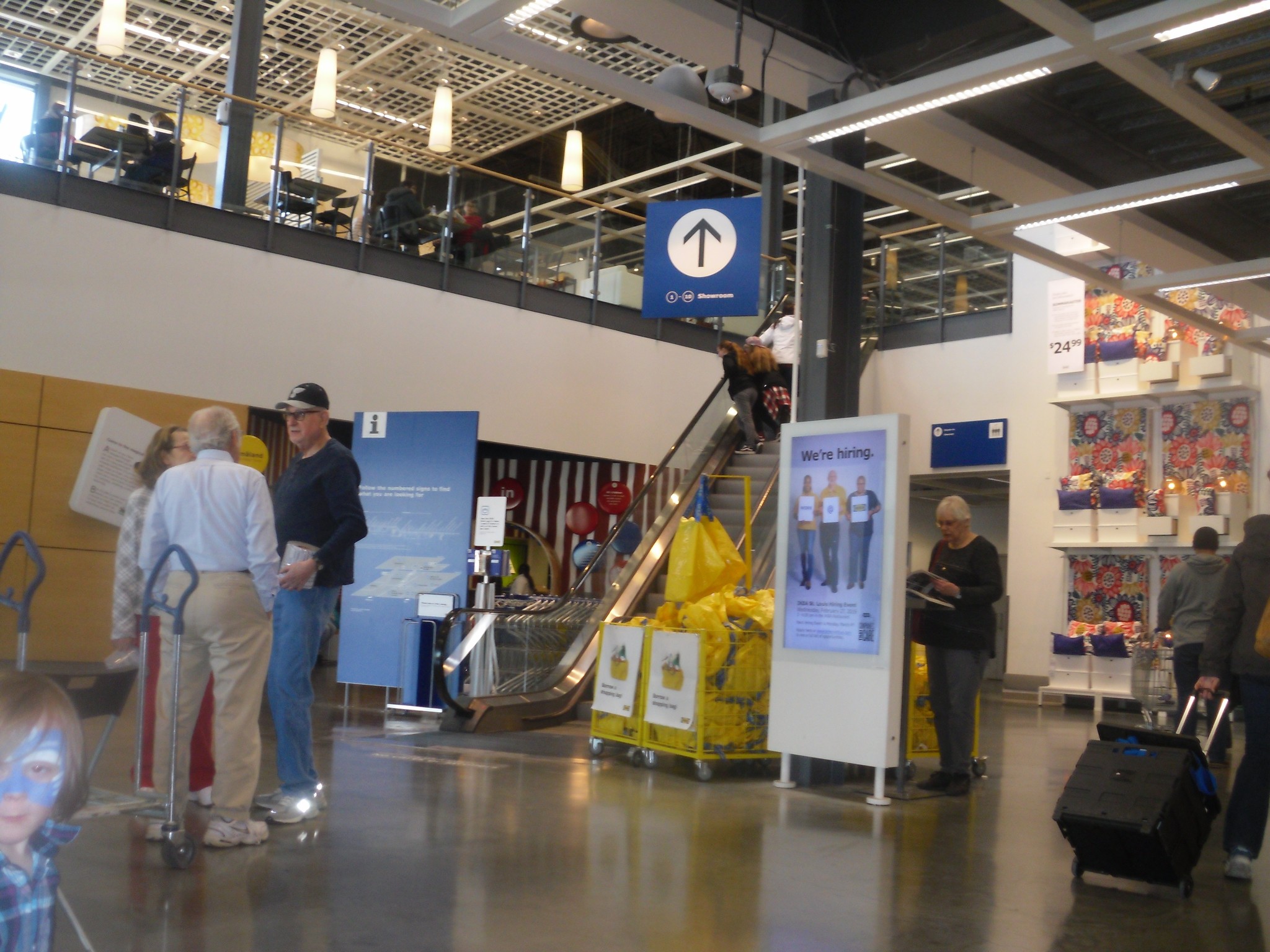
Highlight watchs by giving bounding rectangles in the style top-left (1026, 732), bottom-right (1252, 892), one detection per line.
top-left (954, 587), bottom-right (963, 599)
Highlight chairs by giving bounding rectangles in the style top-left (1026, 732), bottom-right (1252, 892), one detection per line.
top-left (19, 117), bottom-right (577, 293)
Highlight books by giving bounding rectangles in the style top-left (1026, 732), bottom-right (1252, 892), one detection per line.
top-left (906, 570), bottom-right (950, 608)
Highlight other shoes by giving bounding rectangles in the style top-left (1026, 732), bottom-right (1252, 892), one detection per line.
top-left (831, 585), bottom-right (837, 593)
top-left (758, 432), bottom-right (766, 441)
top-left (1224, 854), bottom-right (1251, 879)
top-left (917, 770), bottom-right (951, 790)
top-left (858, 580), bottom-right (863, 589)
top-left (800, 578), bottom-right (807, 586)
top-left (847, 581), bottom-right (854, 589)
top-left (772, 431), bottom-right (781, 442)
top-left (805, 581), bottom-right (811, 589)
top-left (1209, 753), bottom-right (1231, 768)
top-left (946, 773), bottom-right (972, 796)
top-left (821, 579), bottom-right (830, 586)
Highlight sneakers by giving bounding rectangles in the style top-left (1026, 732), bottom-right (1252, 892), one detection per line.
top-left (137, 786), bottom-right (328, 848)
top-left (755, 442), bottom-right (763, 454)
top-left (734, 445), bottom-right (756, 454)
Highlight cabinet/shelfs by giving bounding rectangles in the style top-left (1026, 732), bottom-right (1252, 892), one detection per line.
top-left (1050, 654), bottom-right (1150, 695)
top-left (1053, 508), bottom-right (1149, 542)
top-left (1056, 357), bottom-right (1151, 398)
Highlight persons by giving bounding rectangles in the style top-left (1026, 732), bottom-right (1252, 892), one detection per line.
top-left (0, 669), bottom-right (90, 952)
top-left (915, 495), bottom-right (1003, 796)
top-left (745, 336), bottom-right (791, 441)
top-left (509, 563), bottom-right (534, 595)
top-left (716, 341), bottom-right (763, 454)
top-left (434, 199), bottom-right (483, 267)
top-left (1195, 514), bottom-right (1270, 878)
top-left (1157, 526), bottom-right (1232, 763)
top-left (380, 181), bottom-right (435, 244)
top-left (124, 111), bottom-right (182, 186)
top-left (121, 113), bottom-right (148, 171)
top-left (759, 303), bottom-right (802, 397)
top-left (794, 475), bottom-right (820, 589)
top-left (845, 476), bottom-right (881, 589)
top-left (43, 101), bottom-right (81, 176)
top-left (111, 382), bottom-right (368, 847)
top-left (817, 470), bottom-right (846, 592)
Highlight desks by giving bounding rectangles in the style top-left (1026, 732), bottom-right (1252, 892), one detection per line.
top-left (373, 221), bottom-right (440, 257)
top-left (81, 127), bottom-right (148, 183)
top-left (277, 178), bottom-right (346, 227)
top-left (474, 239), bottom-right (565, 289)
top-left (255, 192), bottom-right (316, 225)
top-left (405, 214), bottom-right (472, 261)
top-left (71, 143), bottom-right (137, 179)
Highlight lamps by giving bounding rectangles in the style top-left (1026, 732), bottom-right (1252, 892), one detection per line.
top-left (561, 46), bottom-right (584, 191)
top-left (428, 36), bottom-right (453, 152)
top-left (310, 0), bottom-right (337, 118)
top-left (1173, 60), bottom-right (1222, 90)
top-left (95, 0), bottom-right (127, 56)
top-left (707, 4), bottom-right (745, 104)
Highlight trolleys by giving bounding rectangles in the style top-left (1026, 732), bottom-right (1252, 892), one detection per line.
top-left (588, 621), bottom-right (988, 784)
top-left (1130, 628), bottom-right (1177, 731)
top-left (0, 530), bottom-right (200, 870)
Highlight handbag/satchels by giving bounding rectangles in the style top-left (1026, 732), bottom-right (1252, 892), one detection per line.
top-left (664, 474), bottom-right (748, 602)
top-left (911, 609), bottom-right (927, 645)
top-left (625, 589), bottom-right (775, 759)
top-left (1254, 599), bottom-right (1270, 659)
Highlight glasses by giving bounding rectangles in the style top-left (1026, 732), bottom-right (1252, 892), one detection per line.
top-left (172, 444), bottom-right (190, 452)
top-left (936, 520), bottom-right (956, 528)
top-left (283, 411), bottom-right (319, 421)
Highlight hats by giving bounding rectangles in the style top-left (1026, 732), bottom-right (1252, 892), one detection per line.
top-left (746, 336), bottom-right (766, 347)
top-left (275, 383), bottom-right (328, 409)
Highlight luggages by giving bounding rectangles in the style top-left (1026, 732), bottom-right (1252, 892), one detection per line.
top-left (1051, 688), bottom-right (1231, 898)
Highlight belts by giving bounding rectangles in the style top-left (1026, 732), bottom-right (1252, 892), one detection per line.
top-left (240, 569), bottom-right (250, 573)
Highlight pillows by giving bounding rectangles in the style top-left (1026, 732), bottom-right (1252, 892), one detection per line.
top-left (1084, 324), bottom-right (1224, 364)
top-left (1050, 619), bottom-right (1144, 658)
top-left (1057, 471), bottom-right (1146, 509)
top-left (1144, 486), bottom-right (1216, 517)
